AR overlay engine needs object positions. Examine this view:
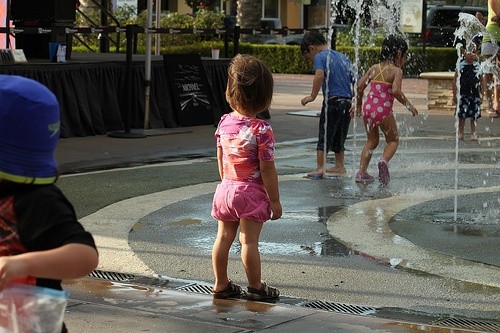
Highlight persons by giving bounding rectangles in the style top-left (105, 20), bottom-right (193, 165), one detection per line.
top-left (481, 0), bottom-right (500, 118)
top-left (210, 54), bottom-right (282, 300)
top-left (0, 73), bottom-right (99, 333)
top-left (356, 34), bottom-right (418, 183)
top-left (453, 41), bottom-right (486, 140)
top-left (300, 30), bottom-right (362, 177)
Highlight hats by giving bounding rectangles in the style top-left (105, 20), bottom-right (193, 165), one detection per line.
top-left (0, 75), bottom-right (61, 185)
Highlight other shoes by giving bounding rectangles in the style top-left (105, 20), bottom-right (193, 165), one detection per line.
top-left (325, 168), bottom-right (346, 176)
top-left (483, 96), bottom-right (488, 101)
top-left (471, 133), bottom-right (478, 141)
top-left (308, 171), bottom-right (323, 180)
top-left (492, 101), bottom-right (500, 111)
top-left (378, 158), bottom-right (390, 184)
top-left (355, 172), bottom-right (374, 181)
top-left (459, 133), bottom-right (464, 140)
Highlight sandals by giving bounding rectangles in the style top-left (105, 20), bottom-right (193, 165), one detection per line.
top-left (247, 282), bottom-right (280, 300)
top-left (213, 279), bottom-right (247, 298)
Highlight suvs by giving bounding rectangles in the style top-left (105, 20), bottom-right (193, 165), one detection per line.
top-left (425, 3), bottom-right (489, 47)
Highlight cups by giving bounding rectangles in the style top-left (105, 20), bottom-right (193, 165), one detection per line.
top-left (212, 48), bottom-right (220, 59)
